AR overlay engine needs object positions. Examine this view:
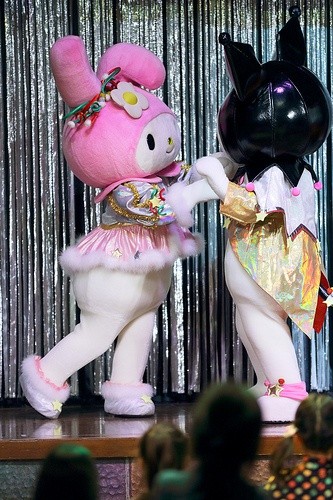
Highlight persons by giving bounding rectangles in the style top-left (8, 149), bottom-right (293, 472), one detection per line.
top-left (133, 382), bottom-right (273, 500)
top-left (263, 393), bottom-right (333, 500)
top-left (33, 445), bottom-right (100, 500)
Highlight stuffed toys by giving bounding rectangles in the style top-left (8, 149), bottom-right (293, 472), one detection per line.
top-left (195, 5), bottom-right (333, 422)
top-left (17, 36), bottom-right (238, 419)
